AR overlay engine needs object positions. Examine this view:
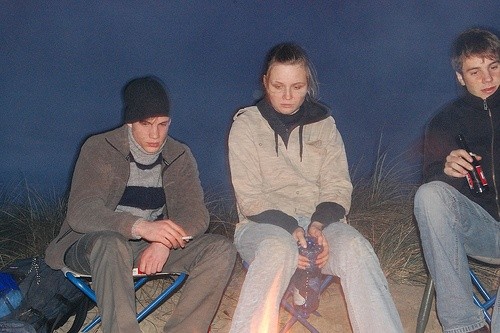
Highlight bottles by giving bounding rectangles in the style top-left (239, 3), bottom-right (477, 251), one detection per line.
top-left (455, 133), bottom-right (490, 197)
top-left (293, 237), bottom-right (323, 311)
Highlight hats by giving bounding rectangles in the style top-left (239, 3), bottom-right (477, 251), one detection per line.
top-left (122, 76), bottom-right (170, 124)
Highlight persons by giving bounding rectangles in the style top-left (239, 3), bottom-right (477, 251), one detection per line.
top-left (414, 26), bottom-right (500, 333)
top-left (44, 76), bottom-right (238, 333)
top-left (229, 41), bottom-right (406, 333)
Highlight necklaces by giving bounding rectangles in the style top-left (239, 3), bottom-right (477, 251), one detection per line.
top-left (274, 107), bottom-right (301, 115)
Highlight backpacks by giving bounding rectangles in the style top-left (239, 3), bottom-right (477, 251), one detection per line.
top-left (0, 254), bottom-right (94, 333)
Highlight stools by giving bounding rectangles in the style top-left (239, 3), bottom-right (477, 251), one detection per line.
top-left (61, 266), bottom-right (186, 333)
top-left (242, 259), bottom-right (333, 333)
top-left (416, 253), bottom-right (500, 333)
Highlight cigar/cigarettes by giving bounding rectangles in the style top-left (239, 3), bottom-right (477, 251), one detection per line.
top-left (182, 236), bottom-right (194, 240)
